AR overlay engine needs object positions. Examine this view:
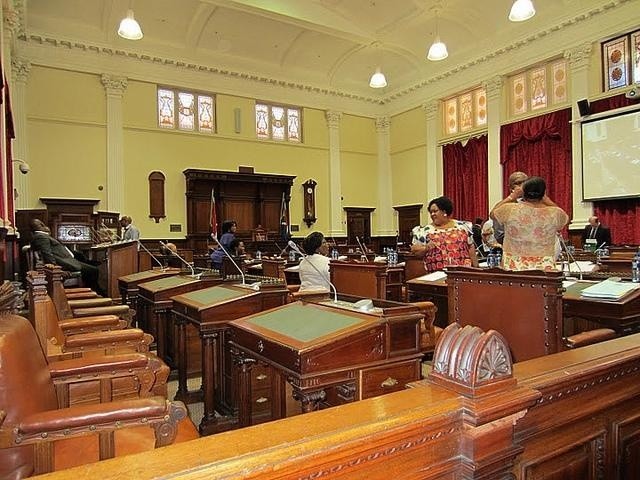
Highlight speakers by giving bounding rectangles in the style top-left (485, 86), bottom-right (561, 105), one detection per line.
top-left (577, 99), bottom-right (590, 117)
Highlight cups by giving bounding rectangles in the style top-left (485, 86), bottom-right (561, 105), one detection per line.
top-left (274, 254), bottom-right (277, 260)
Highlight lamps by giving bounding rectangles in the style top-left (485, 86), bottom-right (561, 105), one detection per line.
top-left (369, 67), bottom-right (387, 87)
top-left (117, 1), bottom-right (144, 41)
top-left (427, 10), bottom-right (449, 61)
top-left (12, 159), bottom-right (29, 174)
top-left (507, 0), bottom-right (536, 22)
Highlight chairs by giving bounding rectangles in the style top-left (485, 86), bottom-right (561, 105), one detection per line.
top-left (442, 263), bottom-right (615, 369)
top-left (330, 263), bottom-right (445, 359)
top-left (261, 256), bottom-right (331, 305)
top-left (221, 253), bottom-right (246, 279)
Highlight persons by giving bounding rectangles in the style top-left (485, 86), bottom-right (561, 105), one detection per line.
top-left (230, 238), bottom-right (246, 256)
top-left (28, 216), bottom-right (98, 289)
top-left (488, 176), bottom-right (570, 273)
top-left (296, 231), bottom-right (333, 293)
top-left (408, 196), bottom-right (479, 272)
top-left (210, 219), bottom-right (238, 270)
top-left (471, 216), bottom-right (484, 252)
top-left (580, 214), bottom-right (611, 249)
top-left (481, 208), bottom-right (500, 254)
top-left (492, 171), bottom-right (530, 246)
top-left (119, 214), bottom-right (141, 250)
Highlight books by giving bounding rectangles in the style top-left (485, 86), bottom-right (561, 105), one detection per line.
top-left (415, 270), bottom-right (447, 282)
top-left (579, 277), bottom-right (640, 305)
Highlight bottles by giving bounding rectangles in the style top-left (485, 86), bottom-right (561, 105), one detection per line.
top-left (595, 249), bottom-right (609, 256)
top-left (567, 246), bottom-right (574, 255)
top-left (487, 250), bottom-right (502, 270)
top-left (384, 247), bottom-right (398, 267)
top-left (632, 256), bottom-right (640, 283)
top-left (584, 244), bottom-right (590, 252)
top-left (289, 250), bottom-right (296, 261)
top-left (332, 249), bottom-right (339, 260)
top-left (256, 250), bottom-right (261, 259)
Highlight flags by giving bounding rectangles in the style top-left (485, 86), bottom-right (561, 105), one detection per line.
top-left (279, 191), bottom-right (289, 242)
top-left (209, 187), bottom-right (217, 240)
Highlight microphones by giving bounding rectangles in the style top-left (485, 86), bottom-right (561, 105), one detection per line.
top-left (288, 241), bottom-right (337, 303)
top-left (159, 241), bottom-right (195, 276)
top-left (84, 223), bottom-right (122, 244)
top-left (211, 233), bottom-right (245, 285)
top-left (355, 235), bottom-right (584, 279)
top-left (135, 236), bottom-right (162, 270)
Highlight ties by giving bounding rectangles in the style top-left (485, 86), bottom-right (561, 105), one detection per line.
top-left (589, 227), bottom-right (596, 240)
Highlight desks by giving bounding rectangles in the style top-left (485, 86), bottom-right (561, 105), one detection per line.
top-left (136, 249), bottom-right (197, 270)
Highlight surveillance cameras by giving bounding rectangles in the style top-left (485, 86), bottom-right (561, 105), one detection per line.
top-left (19, 164), bottom-right (30, 174)
